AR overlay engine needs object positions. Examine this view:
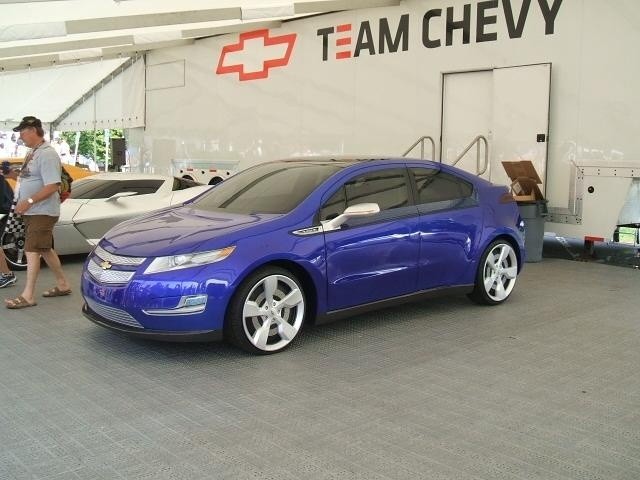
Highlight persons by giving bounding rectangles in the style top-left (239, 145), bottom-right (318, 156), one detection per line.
top-left (0, 174), bottom-right (16, 290)
top-left (5, 116), bottom-right (72, 309)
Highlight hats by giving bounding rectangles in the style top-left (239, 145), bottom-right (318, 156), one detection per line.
top-left (12, 115), bottom-right (42, 133)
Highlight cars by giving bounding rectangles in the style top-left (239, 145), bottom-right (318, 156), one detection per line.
top-left (78, 156), bottom-right (529, 356)
top-left (0, 157), bottom-right (238, 270)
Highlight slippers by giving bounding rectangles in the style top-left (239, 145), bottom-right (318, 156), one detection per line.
top-left (43, 286), bottom-right (73, 297)
top-left (6, 295), bottom-right (37, 309)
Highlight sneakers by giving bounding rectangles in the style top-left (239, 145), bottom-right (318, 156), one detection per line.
top-left (0, 271), bottom-right (18, 288)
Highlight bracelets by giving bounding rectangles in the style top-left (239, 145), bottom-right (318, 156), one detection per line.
top-left (28, 196), bottom-right (34, 206)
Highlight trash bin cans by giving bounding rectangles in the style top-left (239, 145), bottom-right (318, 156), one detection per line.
top-left (517, 205), bottom-right (549, 263)
